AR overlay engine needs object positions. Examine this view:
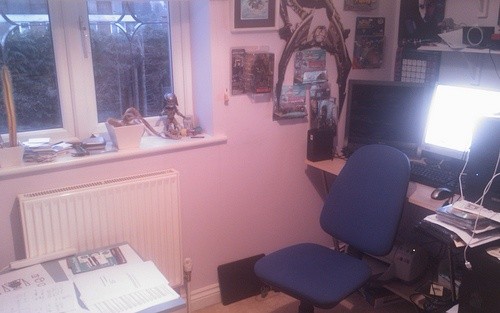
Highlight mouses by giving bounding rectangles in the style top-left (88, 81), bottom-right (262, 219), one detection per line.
top-left (431, 187), bottom-right (453, 200)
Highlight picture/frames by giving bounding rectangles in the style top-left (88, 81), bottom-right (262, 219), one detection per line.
top-left (231, 0), bottom-right (280, 33)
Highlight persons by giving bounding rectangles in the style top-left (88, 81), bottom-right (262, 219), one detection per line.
top-left (160, 93), bottom-right (185, 134)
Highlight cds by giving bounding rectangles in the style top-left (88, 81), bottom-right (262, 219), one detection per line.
top-left (452, 208), bottom-right (485, 219)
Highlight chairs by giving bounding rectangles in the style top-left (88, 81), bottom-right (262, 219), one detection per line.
top-left (255, 144), bottom-right (410, 313)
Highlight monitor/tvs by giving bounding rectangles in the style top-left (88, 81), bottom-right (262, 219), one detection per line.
top-left (419, 81), bottom-right (500, 161)
top-left (344, 79), bottom-right (427, 148)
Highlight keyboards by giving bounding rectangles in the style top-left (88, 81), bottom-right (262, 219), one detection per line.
top-left (409, 160), bottom-right (466, 195)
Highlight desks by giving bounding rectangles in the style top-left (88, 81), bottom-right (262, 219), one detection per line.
top-left (306, 141), bottom-right (500, 313)
top-left (0, 242), bottom-right (186, 313)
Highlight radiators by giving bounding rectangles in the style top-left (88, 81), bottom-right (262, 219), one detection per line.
top-left (18, 169), bottom-right (185, 290)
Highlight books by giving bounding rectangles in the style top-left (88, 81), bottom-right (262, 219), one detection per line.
top-left (422, 200), bottom-right (500, 247)
